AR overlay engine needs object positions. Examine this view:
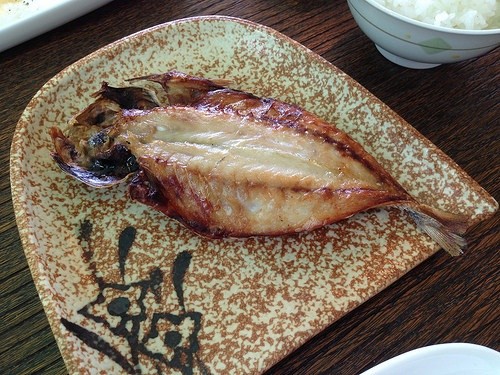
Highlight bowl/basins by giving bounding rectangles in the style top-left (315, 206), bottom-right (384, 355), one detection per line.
top-left (347, 0), bottom-right (500, 69)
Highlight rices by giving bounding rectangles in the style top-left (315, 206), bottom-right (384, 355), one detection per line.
top-left (375, 0), bottom-right (500, 30)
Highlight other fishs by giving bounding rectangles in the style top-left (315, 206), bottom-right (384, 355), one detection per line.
top-left (49, 73), bottom-right (473, 258)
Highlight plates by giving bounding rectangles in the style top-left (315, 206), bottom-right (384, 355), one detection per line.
top-left (0, 0), bottom-right (113, 53)
top-left (360, 343), bottom-right (500, 375)
